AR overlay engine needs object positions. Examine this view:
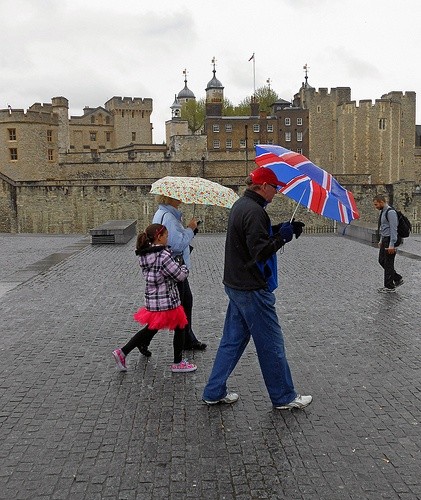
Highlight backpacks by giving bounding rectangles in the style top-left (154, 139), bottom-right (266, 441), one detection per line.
top-left (385, 205), bottom-right (411, 238)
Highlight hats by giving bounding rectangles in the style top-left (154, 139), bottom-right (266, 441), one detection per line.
top-left (248, 166), bottom-right (286, 188)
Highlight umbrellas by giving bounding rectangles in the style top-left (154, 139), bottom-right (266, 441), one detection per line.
top-left (256, 144), bottom-right (360, 254)
top-left (150, 176), bottom-right (241, 233)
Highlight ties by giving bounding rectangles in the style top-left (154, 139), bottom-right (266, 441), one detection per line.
top-left (377, 210), bottom-right (383, 235)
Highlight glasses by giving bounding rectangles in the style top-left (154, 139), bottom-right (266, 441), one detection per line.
top-left (268, 183), bottom-right (279, 191)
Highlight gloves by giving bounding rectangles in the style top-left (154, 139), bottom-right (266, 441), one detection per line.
top-left (291, 218), bottom-right (305, 239)
top-left (280, 221), bottom-right (293, 243)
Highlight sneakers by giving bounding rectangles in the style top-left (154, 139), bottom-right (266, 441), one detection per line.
top-left (377, 287), bottom-right (395, 293)
top-left (393, 278), bottom-right (404, 288)
top-left (201, 393), bottom-right (238, 405)
top-left (274, 395), bottom-right (312, 410)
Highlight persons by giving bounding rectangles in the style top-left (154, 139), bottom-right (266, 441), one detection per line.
top-left (137, 192), bottom-right (207, 358)
top-left (203, 167), bottom-right (313, 410)
top-left (372, 195), bottom-right (404, 293)
top-left (110, 223), bottom-right (200, 372)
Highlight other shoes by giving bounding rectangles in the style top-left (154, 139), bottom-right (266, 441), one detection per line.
top-left (112, 349), bottom-right (126, 370)
top-left (184, 342), bottom-right (206, 351)
top-left (136, 344), bottom-right (151, 357)
top-left (171, 360), bottom-right (196, 372)
